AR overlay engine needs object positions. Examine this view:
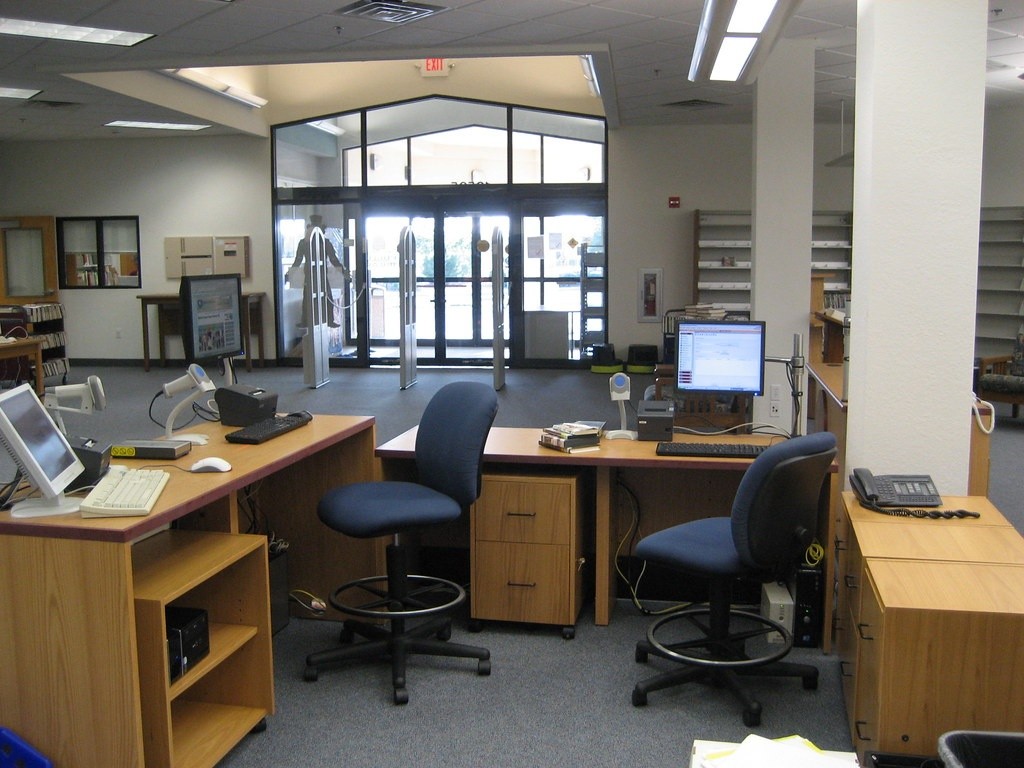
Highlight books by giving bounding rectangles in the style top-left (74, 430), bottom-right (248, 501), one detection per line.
top-left (684, 303), bottom-right (728, 319)
top-left (539, 422), bottom-right (601, 454)
top-left (824, 293), bottom-right (846, 309)
top-left (40, 357), bottom-right (70, 376)
top-left (33, 332), bottom-right (68, 349)
top-left (0, 305), bottom-right (23, 314)
top-left (76, 253), bottom-right (95, 265)
top-left (77, 270), bottom-right (112, 286)
top-left (24, 303), bottom-right (66, 322)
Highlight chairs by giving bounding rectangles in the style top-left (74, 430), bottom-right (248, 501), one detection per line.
top-left (641, 355), bottom-right (749, 433)
top-left (294, 376), bottom-right (504, 709)
top-left (593, 428), bottom-right (847, 731)
top-left (976, 324), bottom-right (1024, 418)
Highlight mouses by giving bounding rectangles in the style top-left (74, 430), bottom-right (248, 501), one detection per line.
top-left (189, 457), bottom-right (232, 472)
top-left (286, 409), bottom-right (313, 420)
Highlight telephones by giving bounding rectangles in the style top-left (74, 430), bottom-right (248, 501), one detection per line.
top-left (849, 468), bottom-right (944, 508)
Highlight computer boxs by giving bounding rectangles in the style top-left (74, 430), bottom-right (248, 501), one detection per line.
top-left (788, 561), bottom-right (822, 646)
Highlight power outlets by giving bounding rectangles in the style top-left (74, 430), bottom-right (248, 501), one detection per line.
top-left (770, 401), bottom-right (783, 417)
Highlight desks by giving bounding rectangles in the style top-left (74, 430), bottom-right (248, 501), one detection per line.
top-left (134, 289), bottom-right (267, 372)
top-left (372, 427), bottom-right (792, 628)
top-left (0, 400), bottom-right (390, 768)
top-left (800, 309), bottom-right (850, 439)
top-left (0, 335), bottom-right (51, 393)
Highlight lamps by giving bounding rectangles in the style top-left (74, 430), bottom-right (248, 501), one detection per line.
top-left (603, 372), bottom-right (639, 440)
top-left (824, 97), bottom-right (853, 166)
top-left (688, 0), bottom-right (804, 94)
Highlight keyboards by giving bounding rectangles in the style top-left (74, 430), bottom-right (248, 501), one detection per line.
top-left (80, 466), bottom-right (170, 518)
top-left (656, 443), bottom-right (772, 459)
top-left (225, 416), bottom-right (307, 444)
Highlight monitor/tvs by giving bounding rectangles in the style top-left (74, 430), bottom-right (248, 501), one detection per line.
top-left (177, 273), bottom-right (245, 365)
top-left (673, 320), bottom-right (766, 397)
top-left (0, 383), bottom-right (86, 517)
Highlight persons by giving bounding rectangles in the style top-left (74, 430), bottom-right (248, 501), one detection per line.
top-left (284, 214), bottom-right (350, 327)
top-left (128, 254), bottom-right (139, 275)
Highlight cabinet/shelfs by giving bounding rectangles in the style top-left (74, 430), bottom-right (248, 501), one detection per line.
top-left (133, 528), bottom-right (280, 768)
top-left (973, 205), bottom-right (1024, 371)
top-left (25, 304), bottom-right (71, 379)
top-left (810, 272), bottom-right (836, 327)
top-left (833, 489), bottom-right (1024, 768)
top-left (693, 209), bottom-right (753, 313)
top-left (812, 209), bottom-right (852, 293)
top-left (469, 463), bottom-right (584, 626)
top-left (968, 403), bottom-right (993, 498)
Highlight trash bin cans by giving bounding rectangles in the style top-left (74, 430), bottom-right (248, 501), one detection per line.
top-left (938, 730), bottom-right (1024, 768)
top-left (269, 553), bottom-right (290, 636)
